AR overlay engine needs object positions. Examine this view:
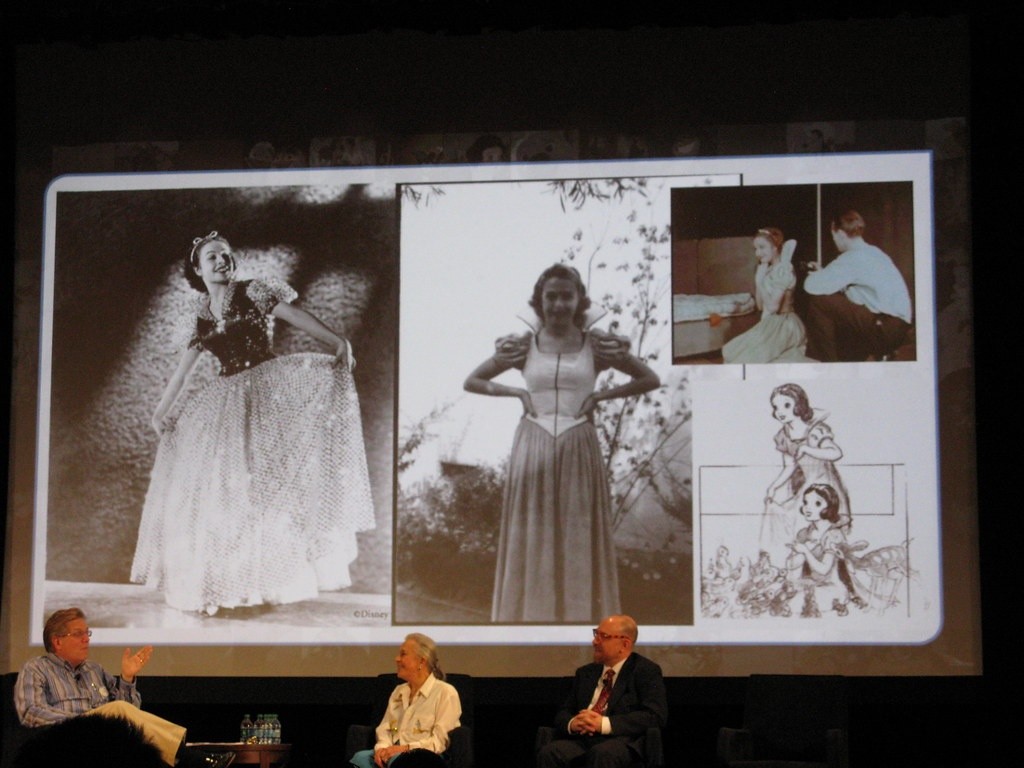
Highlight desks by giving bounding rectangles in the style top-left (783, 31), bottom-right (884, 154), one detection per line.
top-left (187, 742), bottom-right (306, 768)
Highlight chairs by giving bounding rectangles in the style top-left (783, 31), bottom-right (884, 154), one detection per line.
top-left (533, 726), bottom-right (663, 768)
top-left (340, 673), bottom-right (475, 767)
top-left (714, 674), bottom-right (848, 768)
top-left (0, 672), bottom-right (31, 768)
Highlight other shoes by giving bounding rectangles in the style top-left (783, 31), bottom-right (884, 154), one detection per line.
top-left (876, 351), bottom-right (897, 361)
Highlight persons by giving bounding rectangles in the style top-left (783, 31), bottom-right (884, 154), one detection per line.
top-left (722, 210), bottom-right (912, 364)
top-left (375, 632), bottom-right (461, 767)
top-left (130, 230), bottom-right (377, 616)
top-left (536, 614), bottom-right (667, 768)
top-left (14, 607), bottom-right (235, 768)
top-left (464, 264), bottom-right (661, 624)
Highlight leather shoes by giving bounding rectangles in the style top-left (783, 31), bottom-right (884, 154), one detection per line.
top-left (193, 750), bottom-right (236, 768)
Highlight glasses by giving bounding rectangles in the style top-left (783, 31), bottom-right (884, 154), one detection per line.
top-left (593, 629), bottom-right (630, 642)
top-left (56, 630), bottom-right (92, 639)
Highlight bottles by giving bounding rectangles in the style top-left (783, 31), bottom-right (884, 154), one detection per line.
top-left (240, 714), bottom-right (280, 744)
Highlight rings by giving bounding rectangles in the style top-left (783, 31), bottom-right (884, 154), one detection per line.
top-left (140, 660), bottom-right (142, 662)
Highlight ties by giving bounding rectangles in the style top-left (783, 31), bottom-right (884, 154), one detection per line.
top-left (591, 669), bottom-right (615, 716)
